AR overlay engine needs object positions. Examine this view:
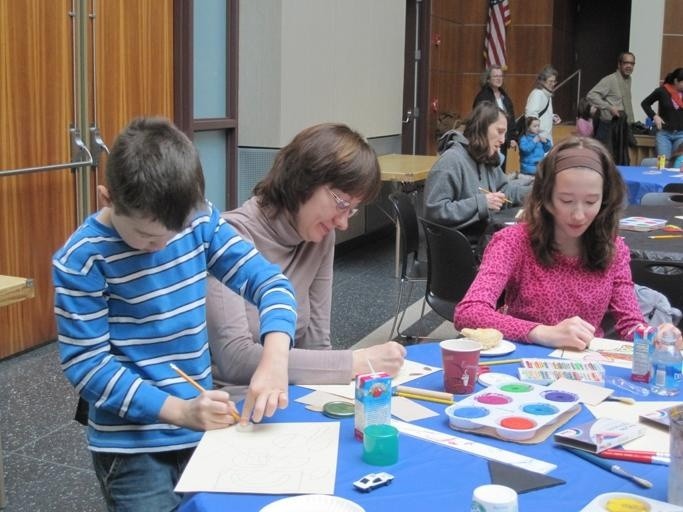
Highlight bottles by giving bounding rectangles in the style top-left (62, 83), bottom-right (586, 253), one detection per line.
top-left (649, 330), bottom-right (682, 396)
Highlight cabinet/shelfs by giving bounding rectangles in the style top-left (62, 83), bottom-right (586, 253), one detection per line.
top-left (1, 1), bottom-right (183, 366)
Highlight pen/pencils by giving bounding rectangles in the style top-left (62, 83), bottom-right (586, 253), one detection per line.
top-left (391, 391), bottom-right (454, 405)
top-left (478, 359), bottom-right (521, 366)
top-left (170, 362), bottom-right (242, 423)
top-left (478, 187), bottom-right (513, 204)
top-left (647, 234), bottom-right (683, 239)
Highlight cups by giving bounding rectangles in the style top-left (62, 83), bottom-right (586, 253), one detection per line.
top-left (437, 339), bottom-right (484, 394)
top-left (472, 485), bottom-right (520, 512)
top-left (668, 405), bottom-right (683, 505)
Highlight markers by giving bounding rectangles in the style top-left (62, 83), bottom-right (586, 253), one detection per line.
top-left (395, 385), bottom-right (455, 400)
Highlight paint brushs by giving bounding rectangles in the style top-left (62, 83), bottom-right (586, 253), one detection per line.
top-left (606, 396), bottom-right (635, 405)
top-left (565, 447), bottom-right (671, 488)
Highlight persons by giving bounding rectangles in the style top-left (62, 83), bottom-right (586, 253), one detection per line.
top-left (422, 97), bottom-right (536, 269)
top-left (472, 50), bottom-right (682, 189)
top-left (50, 115), bottom-right (297, 511)
top-left (454, 133), bottom-right (683, 352)
top-left (205, 123), bottom-right (407, 393)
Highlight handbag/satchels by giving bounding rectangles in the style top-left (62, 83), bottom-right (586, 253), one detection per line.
top-left (516, 115), bottom-right (526, 134)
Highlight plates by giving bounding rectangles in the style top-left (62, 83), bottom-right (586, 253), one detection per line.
top-left (439, 340), bottom-right (516, 357)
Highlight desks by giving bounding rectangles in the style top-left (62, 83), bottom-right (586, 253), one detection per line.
top-left (159, 327), bottom-right (683, 511)
top-left (356, 114), bottom-right (682, 347)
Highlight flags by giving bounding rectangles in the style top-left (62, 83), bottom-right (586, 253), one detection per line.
top-left (482, 0), bottom-right (512, 73)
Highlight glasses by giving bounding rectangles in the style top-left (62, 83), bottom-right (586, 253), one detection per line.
top-left (323, 182), bottom-right (359, 220)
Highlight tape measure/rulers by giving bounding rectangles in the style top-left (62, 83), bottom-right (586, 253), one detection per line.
top-left (486, 459), bottom-right (567, 495)
top-left (389, 419), bottom-right (558, 476)
